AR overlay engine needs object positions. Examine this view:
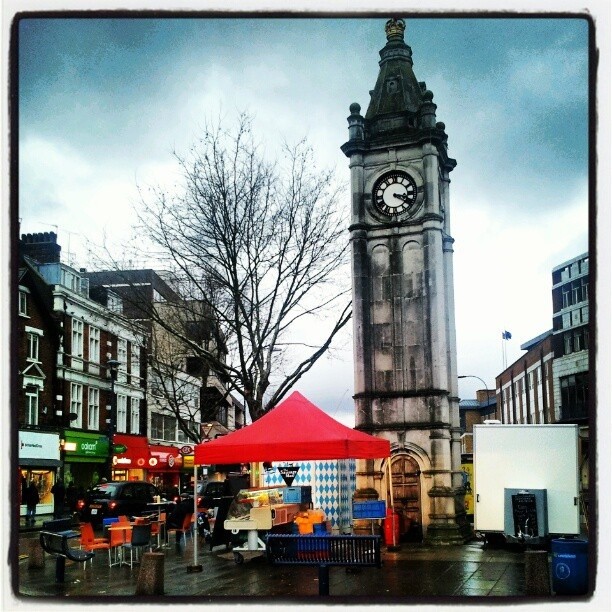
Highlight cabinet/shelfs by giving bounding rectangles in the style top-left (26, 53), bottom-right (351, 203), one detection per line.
top-left (224, 484), bottom-right (310, 551)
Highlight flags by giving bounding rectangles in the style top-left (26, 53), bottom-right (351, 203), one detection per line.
top-left (505, 330), bottom-right (511, 340)
top-left (502, 333), bottom-right (505, 339)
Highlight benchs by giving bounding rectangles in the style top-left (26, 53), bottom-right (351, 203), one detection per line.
top-left (38, 529), bottom-right (92, 584)
top-left (265, 533), bottom-right (380, 594)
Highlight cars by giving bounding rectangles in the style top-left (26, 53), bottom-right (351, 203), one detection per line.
top-left (79, 481), bottom-right (167, 530)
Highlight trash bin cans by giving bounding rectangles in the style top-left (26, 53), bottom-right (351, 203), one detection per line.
top-left (551, 539), bottom-right (589, 594)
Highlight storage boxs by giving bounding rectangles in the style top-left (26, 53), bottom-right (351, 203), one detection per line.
top-left (354, 500), bottom-right (385, 518)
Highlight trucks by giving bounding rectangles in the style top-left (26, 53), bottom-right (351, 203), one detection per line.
top-left (472, 424), bottom-right (582, 550)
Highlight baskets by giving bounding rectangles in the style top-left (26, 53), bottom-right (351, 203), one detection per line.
top-left (292, 523), bottom-right (330, 551)
top-left (353, 501), bottom-right (385, 518)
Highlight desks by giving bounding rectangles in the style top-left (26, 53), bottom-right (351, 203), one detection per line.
top-left (146, 502), bottom-right (177, 552)
top-left (108, 526), bottom-right (134, 567)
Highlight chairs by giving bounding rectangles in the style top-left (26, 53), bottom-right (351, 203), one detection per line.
top-left (103, 517), bottom-right (120, 561)
top-left (79, 525), bottom-right (109, 553)
top-left (166, 515), bottom-right (193, 547)
top-left (119, 516), bottom-right (131, 561)
top-left (109, 522), bottom-right (132, 547)
top-left (152, 513), bottom-right (166, 550)
top-left (120, 524), bottom-right (152, 570)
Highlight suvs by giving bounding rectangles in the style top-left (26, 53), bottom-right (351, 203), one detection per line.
top-left (180, 481), bottom-right (234, 501)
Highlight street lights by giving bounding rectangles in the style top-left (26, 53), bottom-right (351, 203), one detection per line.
top-left (107, 360), bottom-right (120, 481)
top-left (458, 375), bottom-right (490, 419)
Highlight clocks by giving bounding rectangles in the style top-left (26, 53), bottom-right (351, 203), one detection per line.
top-left (372, 169), bottom-right (418, 216)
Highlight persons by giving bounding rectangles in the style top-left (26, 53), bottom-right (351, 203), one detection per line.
top-left (65, 481), bottom-right (79, 516)
top-left (166, 493), bottom-right (195, 529)
top-left (24, 480), bottom-right (42, 527)
top-left (50, 478), bottom-right (66, 520)
